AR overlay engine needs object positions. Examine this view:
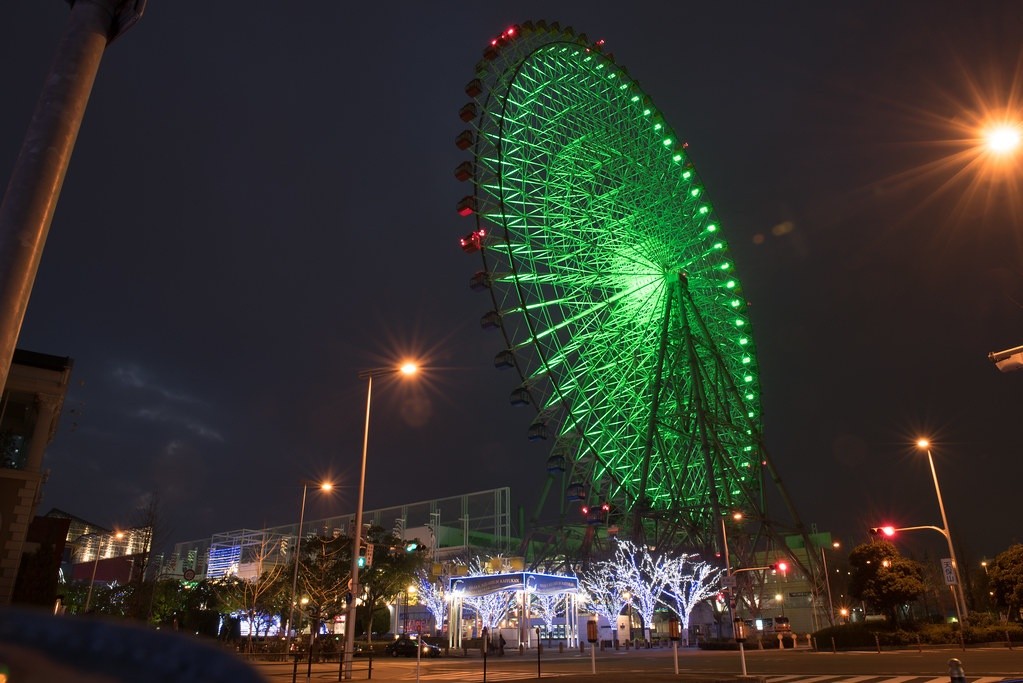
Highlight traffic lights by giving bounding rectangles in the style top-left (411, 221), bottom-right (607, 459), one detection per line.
top-left (769, 562), bottom-right (788, 570)
top-left (870, 527), bottom-right (895, 536)
top-left (582, 503), bottom-right (609, 514)
top-left (406, 542), bottom-right (426, 551)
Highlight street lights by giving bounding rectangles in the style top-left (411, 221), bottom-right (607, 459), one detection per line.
top-left (285, 479), bottom-right (336, 660)
top-left (822, 540), bottom-right (840, 626)
top-left (720, 509), bottom-right (747, 675)
top-left (918, 440), bottom-right (968, 624)
top-left (84, 530), bottom-right (123, 613)
top-left (346, 358), bottom-right (426, 679)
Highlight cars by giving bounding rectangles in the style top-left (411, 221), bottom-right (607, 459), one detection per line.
top-left (385, 637), bottom-right (442, 657)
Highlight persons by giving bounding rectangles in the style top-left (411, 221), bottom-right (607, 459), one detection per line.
top-left (498, 633), bottom-right (505, 655)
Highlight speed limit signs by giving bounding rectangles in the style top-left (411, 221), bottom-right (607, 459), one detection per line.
top-left (185, 569), bottom-right (195, 580)
